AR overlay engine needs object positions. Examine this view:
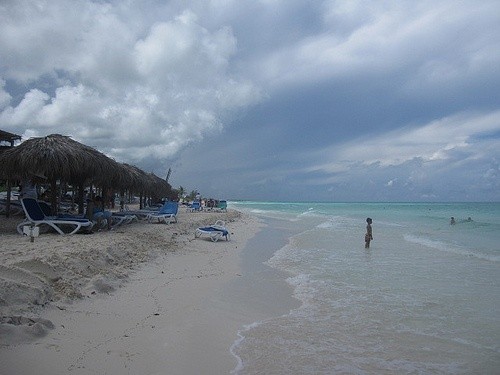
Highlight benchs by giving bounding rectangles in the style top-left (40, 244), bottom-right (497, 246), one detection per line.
top-left (16, 185), bottom-right (95, 235)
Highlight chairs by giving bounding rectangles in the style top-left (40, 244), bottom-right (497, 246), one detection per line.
top-left (112, 202), bottom-right (178, 226)
top-left (189, 199), bottom-right (228, 213)
top-left (194, 220), bottom-right (230, 243)
